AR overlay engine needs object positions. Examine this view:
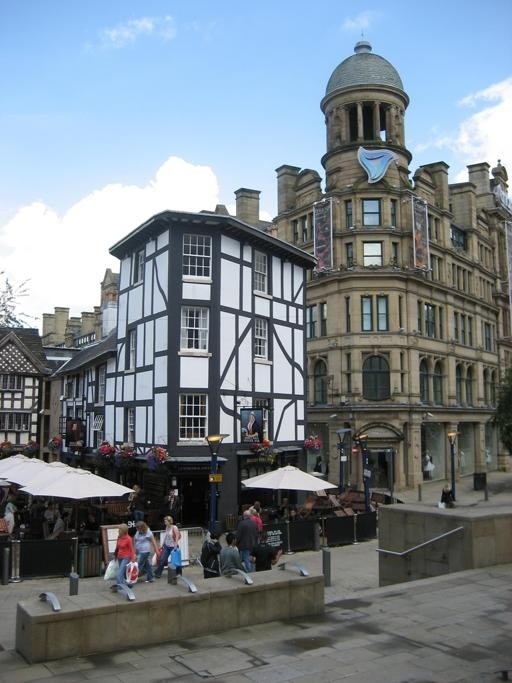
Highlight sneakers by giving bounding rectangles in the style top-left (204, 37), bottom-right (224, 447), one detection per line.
top-left (144, 579), bottom-right (155, 583)
top-left (152, 573), bottom-right (161, 578)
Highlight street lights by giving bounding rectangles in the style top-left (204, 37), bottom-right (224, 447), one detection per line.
top-left (354, 433), bottom-right (370, 511)
top-left (205, 434), bottom-right (227, 531)
top-left (337, 428), bottom-right (348, 494)
top-left (448, 432), bottom-right (458, 500)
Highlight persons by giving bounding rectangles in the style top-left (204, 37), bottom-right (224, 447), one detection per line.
top-left (0, 483), bottom-right (300, 591)
top-left (423, 450), bottom-right (435, 481)
top-left (438, 484), bottom-right (455, 510)
top-left (485, 446), bottom-right (492, 470)
top-left (459, 449), bottom-right (466, 475)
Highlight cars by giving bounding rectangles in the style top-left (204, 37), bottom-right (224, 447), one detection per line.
top-left (460, 448), bottom-right (491, 466)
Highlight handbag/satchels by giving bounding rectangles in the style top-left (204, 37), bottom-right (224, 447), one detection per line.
top-left (113, 546), bottom-right (120, 556)
top-left (103, 559), bottom-right (120, 581)
top-left (125, 561), bottom-right (139, 584)
top-left (170, 548), bottom-right (182, 567)
top-left (172, 527), bottom-right (182, 542)
top-left (151, 547), bottom-right (163, 566)
top-left (438, 502), bottom-right (446, 509)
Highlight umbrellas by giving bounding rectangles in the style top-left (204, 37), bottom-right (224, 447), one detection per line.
top-left (1, 453), bottom-right (136, 574)
top-left (241, 463), bottom-right (339, 555)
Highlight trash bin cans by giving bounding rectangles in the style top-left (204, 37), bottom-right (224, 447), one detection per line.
top-left (225, 513), bottom-right (238, 532)
top-left (79, 543), bottom-right (103, 578)
top-left (473, 472), bottom-right (487, 491)
